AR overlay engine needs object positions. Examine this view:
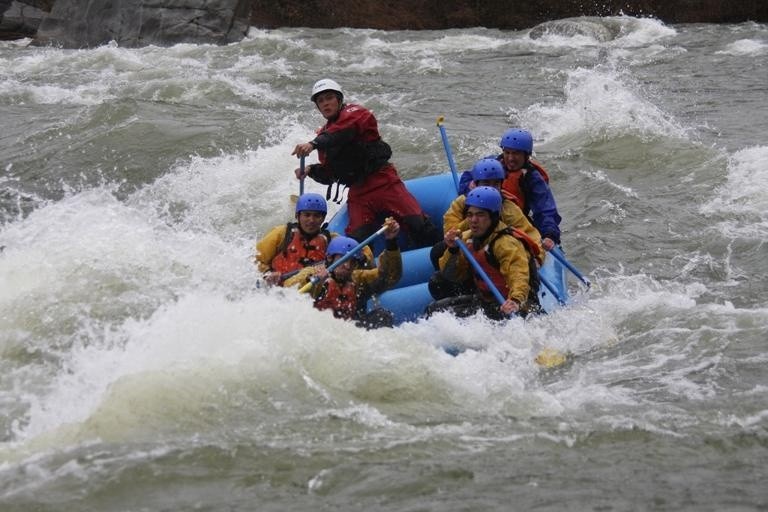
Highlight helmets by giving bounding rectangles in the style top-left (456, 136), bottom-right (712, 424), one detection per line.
top-left (463, 185), bottom-right (503, 214)
top-left (294, 194), bottom-right (327, 220)
top-left (324, 234), bottom-right (363, 261)
top-left (308, 79), bottom-right (344, 98)
top-left (499, 129), bottom-right (533, 154)
top-left (472, 158), bottom-right (505, 182)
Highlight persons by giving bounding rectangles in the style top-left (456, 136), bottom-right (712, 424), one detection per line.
top-left (307, 216), bottom-right (403, 329)
top-left (424, 186), bottom-right (531, 321)
top-left (430, 158), bottom-right (546, 273)
top-left (291, 79), bottom-right (442, 251)
top-left (459, 128), bottom-right (562, 252)
top-left (255, 193), bottom-right (340, 287)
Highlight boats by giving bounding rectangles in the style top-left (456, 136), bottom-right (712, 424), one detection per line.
top-left (312, 168), bottom-right (577, 372)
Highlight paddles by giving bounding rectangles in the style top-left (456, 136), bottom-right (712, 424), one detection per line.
top-left (448, 232), bottom-right (567, 369)
top-left (290, 152), bottom-right (307, 208)
